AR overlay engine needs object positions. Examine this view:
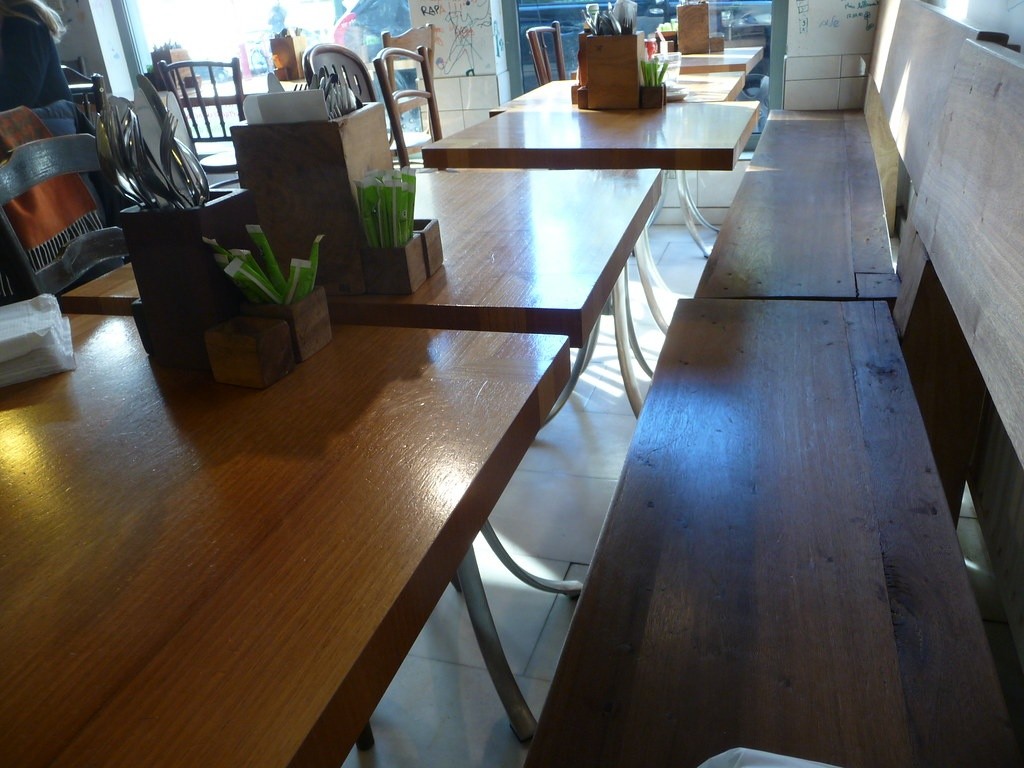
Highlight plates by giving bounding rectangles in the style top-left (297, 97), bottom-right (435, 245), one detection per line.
top-left (665, 92), bottom-right (688, 100)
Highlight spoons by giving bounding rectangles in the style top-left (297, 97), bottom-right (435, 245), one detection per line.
top-left (97, 106), bottom-right (211, 209)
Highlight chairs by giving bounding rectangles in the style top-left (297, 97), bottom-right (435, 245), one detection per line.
top-left (156, 58), bottom-right (252, 175)
top-left (524, 20), bottom-right (566, 87)
top-left (0, 133), bottom-right (129, 307)
top-left (380, 23), bottom-right (440, 165)
top-left (372, 45), bottom-right (444, 174)
top-left (303, 44), bottom-right (380, 102)
top-left (60, 57), bottom-right (113, 125)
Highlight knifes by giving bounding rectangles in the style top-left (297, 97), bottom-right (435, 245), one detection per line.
top-left (137, 74), bottom-right (200, 206)
top-left (301, 55), bottom-right (314, 89)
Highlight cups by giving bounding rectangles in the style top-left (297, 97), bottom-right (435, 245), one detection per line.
top-left (652, 52), bottom-right (682, 86)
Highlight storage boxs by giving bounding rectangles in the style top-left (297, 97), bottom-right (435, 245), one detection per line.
top-left (361, 232), bottom-right (427, 295)
top-left (228, 100), bottom-right (396, 294)
top-left (412, 219), bottom-right (445, 277)
top-left (151, 46), bottom-right (191, 92)
top-left (570, 2), bottom-right (724, 109)
top-left (203, 314), bottom-right (295, 390)
top-left (118, 184), bottom-right (258, 370)
top-left (270, 34), bottom-right (311, 81)
top-left (241, 284), bottom-right (334, 363)
top-left (130, 298), bottom-right (153, 355)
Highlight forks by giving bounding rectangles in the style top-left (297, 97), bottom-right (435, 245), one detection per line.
top-left (160, 112), bottom-right (196, 209)
top-left (620, 18), bottom-right (633, 34)
top-left (333, 84), bottom-right (350, 115)
top-left (328, 107), bottom-right (340, 121)
top-left (292, 84), bottom-right (308, 92)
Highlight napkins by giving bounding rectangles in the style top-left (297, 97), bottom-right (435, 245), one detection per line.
top-left (0, 293), bottom-right (74, 387)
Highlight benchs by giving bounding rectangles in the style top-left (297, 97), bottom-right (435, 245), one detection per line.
top-left (519, 37), bottom-right (1024, 767)
top-left (696, 0), bottom-right (1012, 316)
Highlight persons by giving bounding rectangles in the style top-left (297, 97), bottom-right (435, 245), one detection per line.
top-left (0, 0), bottom-right (126, 297)
top-left (269, 5), bottom-right (288, 52)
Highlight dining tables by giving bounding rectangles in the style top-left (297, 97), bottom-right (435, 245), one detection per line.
top-left (420, 101), bottom-right (762, 428)
top-left (63, 165), bottom-right (677, 600)
top-left (0, 316), bottom-right (569, 767)
top-left (173, 75), bottom-right (308, 108)
top-left (485, 71), bottom-right (746, 337)
top-left (569, 44), bottom-right (768, 260)
